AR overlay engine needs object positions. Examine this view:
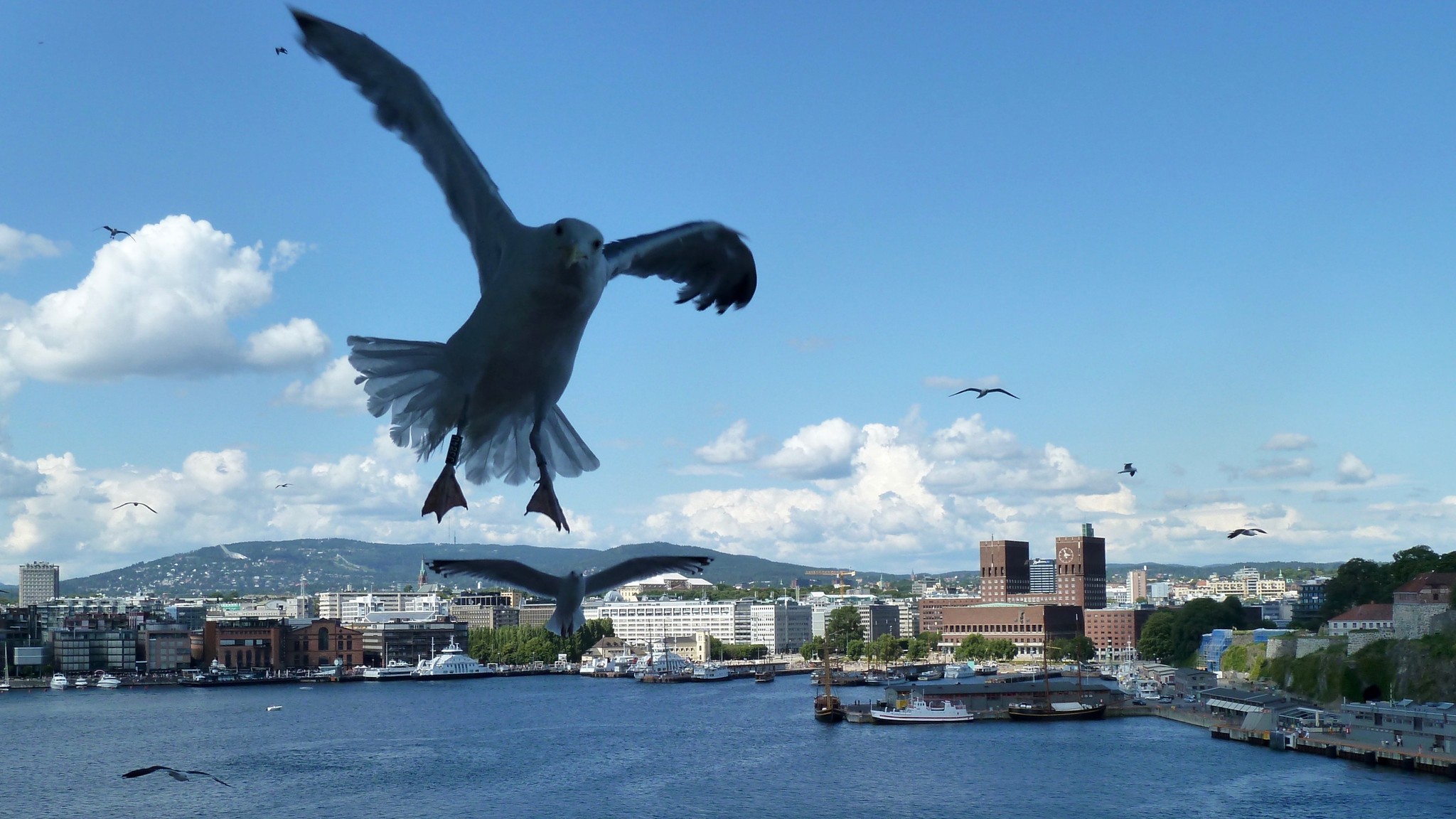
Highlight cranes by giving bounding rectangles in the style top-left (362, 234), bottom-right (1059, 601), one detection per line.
top-left (805, 570), bottom-right (856, 600)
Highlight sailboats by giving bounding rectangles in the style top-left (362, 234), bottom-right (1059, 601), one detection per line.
top-left (0, 641), bottom-right (11, 691)
top-left (813, 630), bottom-right (848, 723)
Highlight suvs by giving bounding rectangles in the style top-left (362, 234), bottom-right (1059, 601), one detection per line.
top-left (1132, 699), bottom-right (1146, 706)
top-left (1144, 694), bottom-right (1160, 700)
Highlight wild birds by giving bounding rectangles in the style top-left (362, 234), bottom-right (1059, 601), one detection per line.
top-left (1118, 463), bottom-right (1137, 477)
top-left (949, 388), bottom-right (1020, 400)
top-left (275, 46), bottom-right (288, 55)
top-left (112, 502), bottom-right (158, 514)
top-left (93, 226), bottom-right (137, 243)
top-left (424, 555), bottom-right (714, 638)
top-left (121, 766), bottom-right (233, 788)
top-left (286, 4), bottom-right (758, 535)
top-left (1227, 529), bottom-right (1268, 540)
top-left (275, 484), bottom-right (293, 488)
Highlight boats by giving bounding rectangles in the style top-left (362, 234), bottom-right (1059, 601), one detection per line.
top-left (300, 687), bottom-right (313, 690)
top-left (50, 671), bottom-right (67, 689)
top-left (1100, 635), bottom-right (1159, 698)
top-left (266, 706), bottom-right (283, 711)
top-left (870, 689), bottom-right (980, 725)
top-left (96, 674), bottom-right (121, 689)
top-left (75, 675), bottom-right (88, 689)
top-left (625, 634), bottom-right (732, 682)
top-left (1014, 658), bottom-right (1040, 674)
top-left (755, 642), bottom-right (775, 683)
top-left (362, 634), bottom-right (494, 682)
top-left (865, 655), bottom-right (998, 686)
top-left (1008, 642), bottom-right (1106, 720)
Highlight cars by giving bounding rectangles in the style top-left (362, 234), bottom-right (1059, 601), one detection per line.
top-left (1183, 697), bottom-right (1195, 703)
top-left (1062, 660), bottom-right (1102, 672)
top-left (1158, 695), bottom-right (1174, 704)
